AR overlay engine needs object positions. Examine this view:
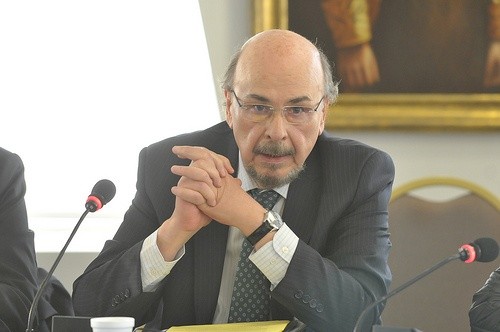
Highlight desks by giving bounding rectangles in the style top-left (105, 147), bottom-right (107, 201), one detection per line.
top-left (27, 216), bottom-right (121, 297)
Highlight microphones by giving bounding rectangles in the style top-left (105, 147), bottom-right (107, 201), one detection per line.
top-left (25, 179), bottom-right (116, 332)
top-left (353, 237), bottom-right (500, 332)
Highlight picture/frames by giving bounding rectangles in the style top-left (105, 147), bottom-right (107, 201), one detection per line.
top-left (253, 0), bottom-right (500, 130)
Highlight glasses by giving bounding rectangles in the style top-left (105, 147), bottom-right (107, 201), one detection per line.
top-left (230, 89), bottom-right (326, 125)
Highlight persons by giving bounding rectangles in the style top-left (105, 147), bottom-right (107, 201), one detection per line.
top-left (0, 146), bottom-right (40, 332)
top-left (71, 29), bottom-right (396, 332)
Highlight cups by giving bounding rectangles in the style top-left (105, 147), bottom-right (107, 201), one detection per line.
top-left (90, 317), bottom-right (134, 332)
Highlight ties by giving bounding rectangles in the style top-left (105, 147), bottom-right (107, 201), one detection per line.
top-left (227, 187), bottom-right (284, 323)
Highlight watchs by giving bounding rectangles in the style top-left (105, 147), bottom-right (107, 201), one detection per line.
top-left (247, 209), bottom-right (284, 245)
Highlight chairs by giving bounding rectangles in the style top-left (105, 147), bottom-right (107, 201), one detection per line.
top-left (381, 176), bottom-right (500, 332)
